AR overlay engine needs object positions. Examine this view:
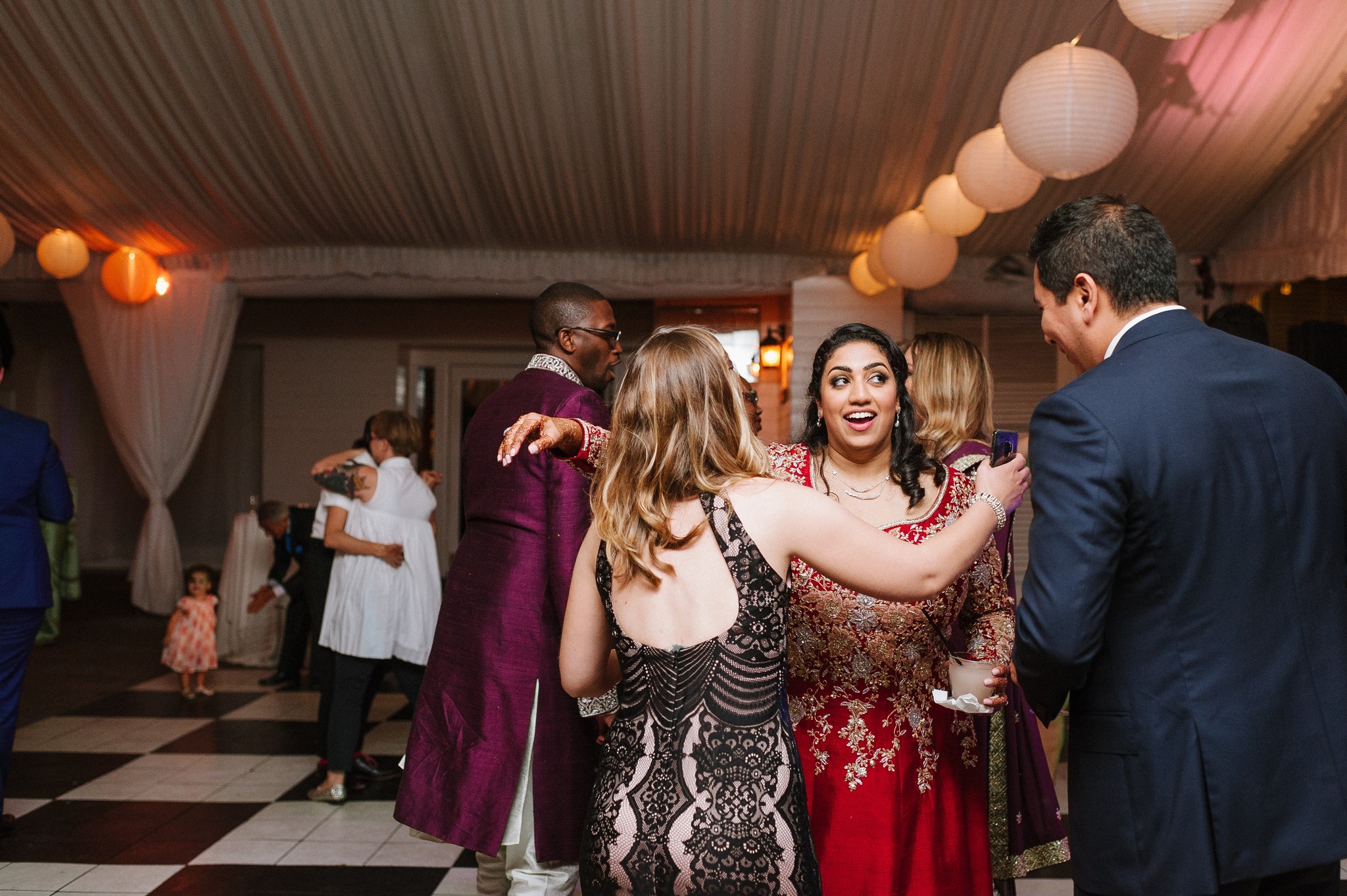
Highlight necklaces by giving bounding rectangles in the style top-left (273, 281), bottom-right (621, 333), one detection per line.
top-left (826, 445), bottom-right (893, 502)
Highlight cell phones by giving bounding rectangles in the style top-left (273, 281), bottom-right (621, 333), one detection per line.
top-left (989, 429), bottom-right (1020, 469)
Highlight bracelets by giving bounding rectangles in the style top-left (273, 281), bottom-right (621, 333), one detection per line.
top-left (965, 493), bottom-right (1007, 533)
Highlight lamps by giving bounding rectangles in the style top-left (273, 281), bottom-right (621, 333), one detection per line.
top-left (748, 353), bottom-right (760, 380)
top-left (759, 321), bottom-right (786, 369)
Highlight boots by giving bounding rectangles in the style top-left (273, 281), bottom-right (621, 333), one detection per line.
top-left (476, 843), bottom-right (512, 896)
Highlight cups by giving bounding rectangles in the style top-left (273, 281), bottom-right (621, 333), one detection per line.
top-left (946, 651), bottom-right (999, 708)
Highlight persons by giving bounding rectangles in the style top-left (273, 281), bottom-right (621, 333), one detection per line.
top-left (493, 321), bottom-right (1030, 896)
top-left (375, 276), bottom-right (628, 894)
top-left (1, 361), bottom-right (78, 831)
top-left (1203, 301), bottom-right (1270, 351)
top-left (291, 414), bottom-right (443, 721)
top-left (1284, 319), bottom-right (1347, 396)
top-left (160, 562), bottom-right (220, 700)
top-left (558, 319), bottom-right (1025, 896)
top-left (961, 193), bottom-right (1347, 896)
top-left (866, 336), bottom-right (1072, 894)
top-left (246, 498), bottom-right (336, 693)
top-left (307, 409), bottom-right (443, 805)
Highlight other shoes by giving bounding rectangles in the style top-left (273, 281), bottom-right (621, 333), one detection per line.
top-left (194, 685), bottom-right (215, 696)
top-left (0, 813), bottom-right (17, 834)
top-left (257, 672), bottom-right (300, 686)
top-left (276, 673), bottom-right (322, 692)
top-left (307, 782), bottom-right (347, 802)
top-left (182, 688), bottom-right (196, 699)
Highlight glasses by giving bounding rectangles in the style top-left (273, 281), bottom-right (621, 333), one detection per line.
top-left (555, 326), bottom-right (622, 349)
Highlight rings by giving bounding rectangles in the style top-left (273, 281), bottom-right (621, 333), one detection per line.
top-left (503, 426), bottom-right (511, 436)
top-left (1005, 701), bottom-right (1009, 706)
